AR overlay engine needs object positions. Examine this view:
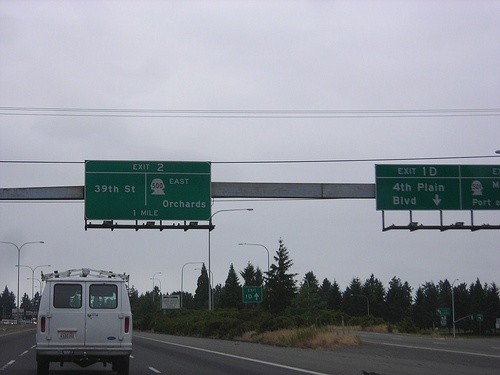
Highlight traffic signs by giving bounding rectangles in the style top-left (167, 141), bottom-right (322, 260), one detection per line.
top-left (241, 286), bottom-right (263, 304)
top-left (373, 164), bottom-right (500, 212)
top-left (84, 159), bottom-right (213, 221)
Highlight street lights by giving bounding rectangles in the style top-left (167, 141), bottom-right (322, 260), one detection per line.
top-left (27, 277), bottom-right (46, 296)
top-left (443, 278), bottom-right (459, 336)
top-left (209, 208), bottom-right (255, 310)
top-left (181, 262), bottom-right (204, 306)
top-left (238, 242), bottom-right (270, 284)
top-left (0, 241), bottom-right (45, 319)
top-left (17, 265), bottom-right (52, 302)
top-left (150, 272), bottom-right (161, 295)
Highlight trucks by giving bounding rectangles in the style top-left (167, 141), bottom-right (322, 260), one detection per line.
top-left (35, 267), bottom-right (133, 375)
